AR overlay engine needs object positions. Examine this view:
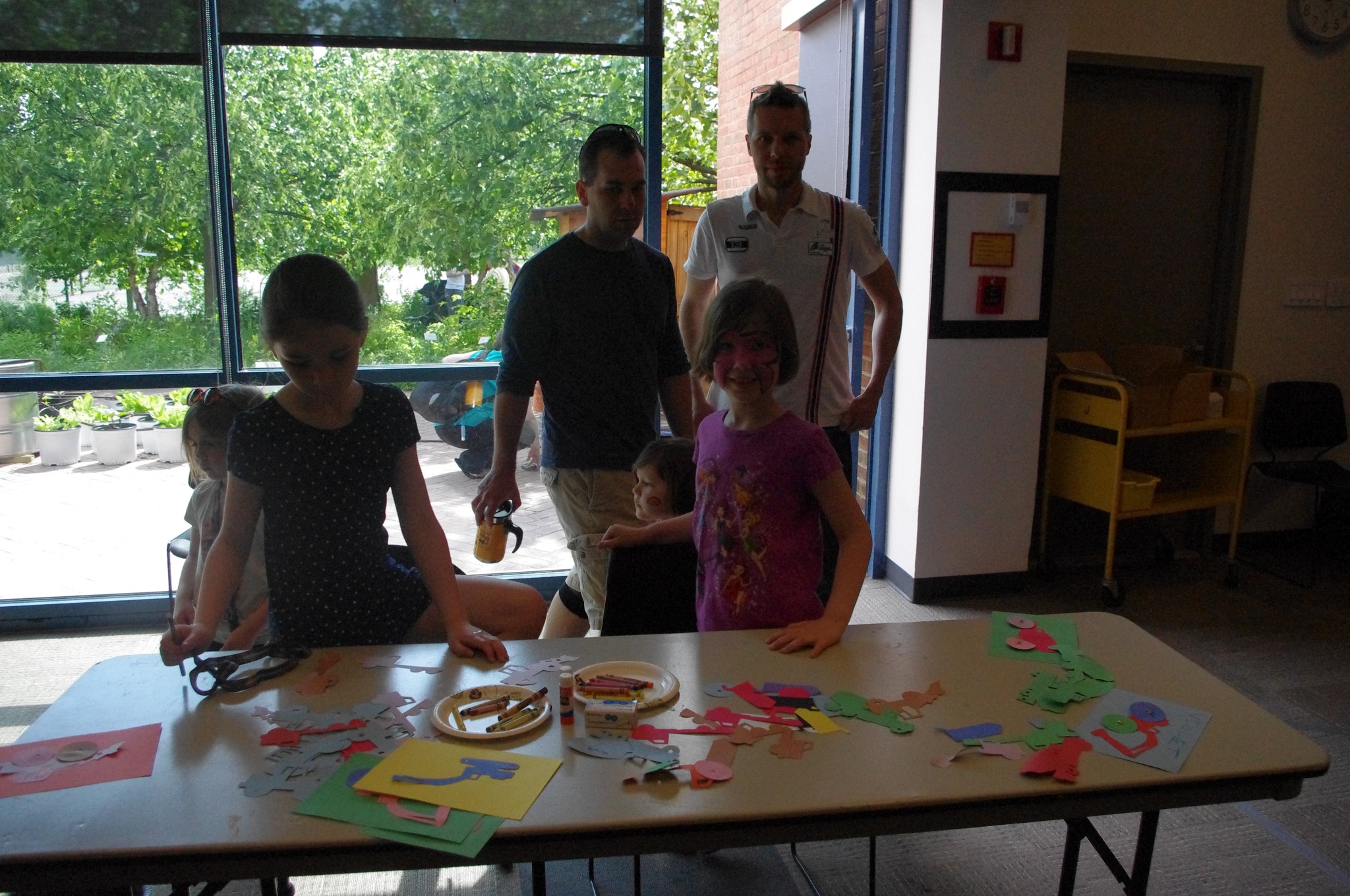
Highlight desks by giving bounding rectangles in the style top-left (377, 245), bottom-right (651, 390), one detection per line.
top-left (0, 609), bottom-right (1332, 894)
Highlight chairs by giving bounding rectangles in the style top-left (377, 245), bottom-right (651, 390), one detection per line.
top-left (588, 536), bottom-right (713, 895)
top-left (1242, 381), bottom-right (1348, 569)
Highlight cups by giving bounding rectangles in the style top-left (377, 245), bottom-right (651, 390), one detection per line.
top-left (473, 500), bottom-right (523, 565)
top-left (465, 380), bottom-right (483, 410)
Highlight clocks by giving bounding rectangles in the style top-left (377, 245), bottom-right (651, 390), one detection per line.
top-left (1286, 1), bottom-right (1350, 52)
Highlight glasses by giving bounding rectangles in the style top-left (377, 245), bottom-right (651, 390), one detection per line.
top-left (749, 84), bottom-right (808, 112)
top-left (186, 387), bottom-right (243, 413)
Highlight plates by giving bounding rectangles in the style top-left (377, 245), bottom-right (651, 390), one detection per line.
top-left (573, 661), bottom-right (680, 709)
top-left (430, 684), bottom-right (552, 739)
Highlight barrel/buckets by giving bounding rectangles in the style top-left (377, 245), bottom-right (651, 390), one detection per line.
top-left (0, 358), bottom-right (40, 459)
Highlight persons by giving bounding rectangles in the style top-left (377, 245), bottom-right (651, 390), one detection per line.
top-left (596, 277), bottom-right (872, 657)
top-left (169, 387), bottom-right (274, 652)
top-left (431, 333), bottom-right (525, 481)
top-left (469, 122), bottom-right (698, 639)
top-left (604, 435), bottom-right (701, 637)
top-left (525, 373), bottom-right (557, 473)
top-left (680, 80), bottom-right (903, 615)
top-left (161, 252), bottom-right (510, 667)
top-left (415, 244), bottom-right (530, 328)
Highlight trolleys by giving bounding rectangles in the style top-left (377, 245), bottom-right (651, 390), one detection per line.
top-left (1032, 366), bottom-right (1257, 610)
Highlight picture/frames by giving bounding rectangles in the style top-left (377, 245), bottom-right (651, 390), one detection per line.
top-left (926, 170), bottom-right (1059, 343)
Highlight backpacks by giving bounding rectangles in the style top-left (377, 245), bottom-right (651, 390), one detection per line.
top-left (409, 349), bottom-right (496, 424)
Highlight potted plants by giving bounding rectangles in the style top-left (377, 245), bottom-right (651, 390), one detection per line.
top-left (143, 389), bottom-right (199, 466)
top-left (28, 393), bottom-right (93, 467)
top-left (82, 389), bottom-right (152, 467)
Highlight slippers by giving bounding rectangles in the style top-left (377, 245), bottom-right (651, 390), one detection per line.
top-left (454, 457), bottom-right (484, 479)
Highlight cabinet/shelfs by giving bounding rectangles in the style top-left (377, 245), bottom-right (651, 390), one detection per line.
top-left (1037, 357), bottom-right (1254, 586)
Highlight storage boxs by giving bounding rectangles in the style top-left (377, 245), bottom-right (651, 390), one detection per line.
top-left (1062, 344), bottom-right (1170, 434)
top-left (1126, 343), bottom-right (1212, 424)
top-left (1117, 467), bottom-right (1162, 512)
